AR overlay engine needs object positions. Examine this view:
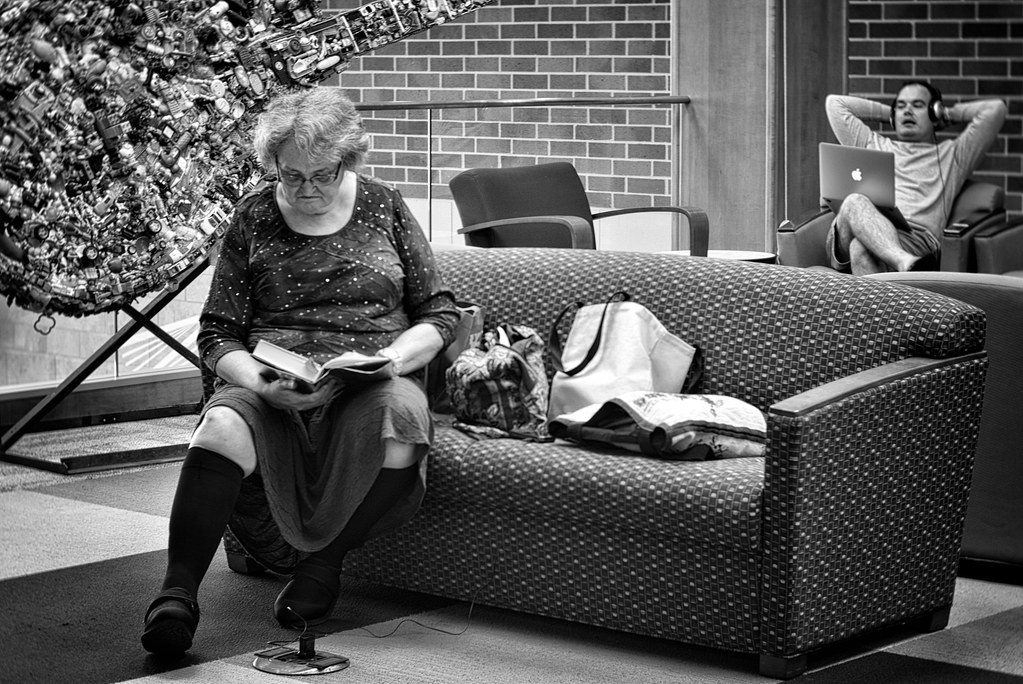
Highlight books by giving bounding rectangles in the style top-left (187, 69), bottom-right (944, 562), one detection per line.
top-left (249, 339), bottom-right (393, 392)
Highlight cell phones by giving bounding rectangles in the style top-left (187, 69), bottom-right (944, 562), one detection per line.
top-left (943, 222), bottom-right (969, 234)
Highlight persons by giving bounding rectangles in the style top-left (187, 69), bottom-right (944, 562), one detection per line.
top-left (825, 81), bottom-right (1008, 277)
top-left (140, 88), bottom-right (462, 655)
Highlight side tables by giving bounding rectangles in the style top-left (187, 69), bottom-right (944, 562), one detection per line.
top-left (657, 247), bottom-right (775, 265)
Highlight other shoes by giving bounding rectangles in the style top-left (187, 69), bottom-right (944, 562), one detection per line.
top-left (903, 251), bottom-right (938, 272)
top-left (272, 556), bottom-right (342, 630)
top-left (140, 586), bottom-right (203, 655)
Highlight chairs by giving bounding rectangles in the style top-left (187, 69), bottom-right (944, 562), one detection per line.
top-left (442, 159), bottom-right (714, 257)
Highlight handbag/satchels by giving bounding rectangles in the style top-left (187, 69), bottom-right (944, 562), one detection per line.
top-left (444, 321), bottom-right (555, 445)
top-left (421, 297), bottom-right (487, 416)
top-left (544, 288), bottom-right (705, 423)
top-left (545, 393), bottom-right (767, 461)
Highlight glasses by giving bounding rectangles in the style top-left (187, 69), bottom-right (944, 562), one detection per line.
top-left (273, 153), bottom-right (343, 188)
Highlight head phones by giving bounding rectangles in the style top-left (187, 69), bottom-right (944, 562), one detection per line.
top-left (889, 83), bottom-right (943, 131)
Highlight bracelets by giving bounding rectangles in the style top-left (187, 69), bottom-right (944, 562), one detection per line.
top-left (945, 107), bottom-right (950, 119)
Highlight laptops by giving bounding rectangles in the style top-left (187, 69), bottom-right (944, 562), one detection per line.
top-left (819, 142), bottom-right (913, 234)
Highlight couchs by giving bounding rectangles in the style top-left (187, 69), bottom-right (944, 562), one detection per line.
top-left (853, 266), bottom-right (1023, 582)
top-left (776, 177), bottom-right (1006, 271)
top-left (202, 245), bottom-right (999, 674)
top-left (975, 214), bottom-right (1023, 278)
